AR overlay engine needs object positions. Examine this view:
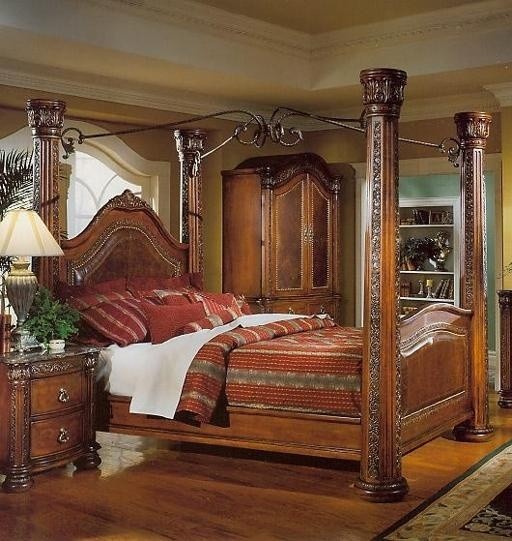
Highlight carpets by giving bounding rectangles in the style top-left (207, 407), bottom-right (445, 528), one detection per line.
top-left (371, 440), bottom-right (512, 541)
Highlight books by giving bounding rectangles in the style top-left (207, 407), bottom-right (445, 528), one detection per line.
top-left (400, 209), bottom-right (428, 225)
top-left (400, 282), bottom-right (412, 298)
top-left (400, 306), bottom-right (418, 314)
top-left (433, 279), bottom-right (454, 300)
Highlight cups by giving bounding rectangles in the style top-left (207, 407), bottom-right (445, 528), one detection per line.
top-left (48, 340), bottom-right (66, 351)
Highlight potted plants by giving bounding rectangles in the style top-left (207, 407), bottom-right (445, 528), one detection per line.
top-left (21, 285), bottom-right (80, 349)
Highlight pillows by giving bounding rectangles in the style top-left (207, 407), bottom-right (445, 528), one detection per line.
top-left (69, 272), bottom-right (242, 347)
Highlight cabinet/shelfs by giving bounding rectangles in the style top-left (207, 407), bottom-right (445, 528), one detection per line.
top-left (221, 151), bottom-right (343, 326)
top-left (399, 196), bottom-right (460, 319)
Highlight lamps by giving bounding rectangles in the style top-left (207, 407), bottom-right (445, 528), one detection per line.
top-left (0, 211), bottom-right (65, 336)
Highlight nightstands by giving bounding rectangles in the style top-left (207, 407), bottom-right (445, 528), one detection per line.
top-left (0, 349), bottom-right (101, 492)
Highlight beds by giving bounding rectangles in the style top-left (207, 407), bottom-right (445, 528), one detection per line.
top-left (25, 68), bottom-right (493, 503)
